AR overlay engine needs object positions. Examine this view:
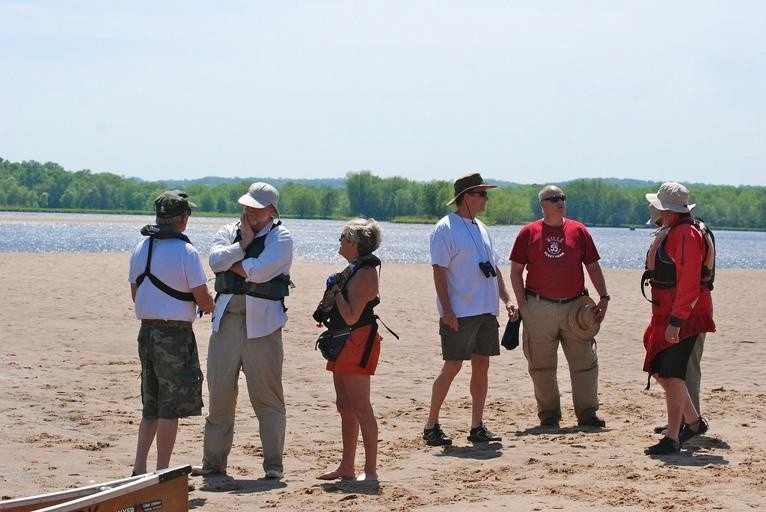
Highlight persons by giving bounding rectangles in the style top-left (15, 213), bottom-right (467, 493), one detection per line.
top-left (127, 188), bottom-right (215, 493)
top-left (643, 180), bottom-right (717, 456)
top-left (508, 183), bottom-right (611, 432)
top-left (422, 172), bottom-right (520, 448)
top-left (189, 181), bottom-right (293, 480)
top-left (309, 216), bottom-right (382, 484)
top-left (645, 202), bottom-right (715, 445)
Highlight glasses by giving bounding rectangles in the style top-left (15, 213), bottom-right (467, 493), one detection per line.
top-left (341, 234), bottom-right (344, 238)
top-left (541, 195), bottom-right (566, 202)
top-left (468, 191), bottom-right (487, 198)
top-left (446, 173), bottom-right (498, 206)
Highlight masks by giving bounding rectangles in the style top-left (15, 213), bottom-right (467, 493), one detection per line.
top-left (645, 204), bottom-right (662, 226)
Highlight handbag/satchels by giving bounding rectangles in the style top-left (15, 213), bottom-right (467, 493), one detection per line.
top-left (501, 307), bottom-right (522, 351)
top-left (318, 326), bottom-right (352, 361)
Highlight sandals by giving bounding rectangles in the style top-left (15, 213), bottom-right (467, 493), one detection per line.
top-left (644, 436), bottom-right (680, 456)
top-left (677, 415), bottom-right (709, 443)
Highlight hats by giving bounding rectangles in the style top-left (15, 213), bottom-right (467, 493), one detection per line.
top-left (154, 190), bottom-right (198, 218)
top-left (568, 295), bottom-right (600, 341)
top-left (645, 182), bottom-right (696, 214)
top-left (238, 182), bottom-right (280, 218)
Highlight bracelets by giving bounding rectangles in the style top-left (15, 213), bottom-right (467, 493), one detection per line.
top-left (598, 293), bottom-right (610, 302)
top-left (333, 288), bottom-right (344, 298)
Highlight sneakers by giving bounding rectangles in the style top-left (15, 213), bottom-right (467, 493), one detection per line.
top-left (467, 425), bottom-right (502, 442)
top-left (190, 465), bottom-right (227, 476)
top-left (540, 421), bottom-right (560, 429)
top-left (423, 423), bottom-right (452, 445)
top-left (654, 424), bottom-right (669, 433)
top-left (265, 470), bottom-right (283, 478)
top-left (578, 419), bottom-right (605, 427)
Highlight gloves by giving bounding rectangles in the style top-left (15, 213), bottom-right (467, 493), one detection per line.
top-left (326, 273), bottom-right (337, 289)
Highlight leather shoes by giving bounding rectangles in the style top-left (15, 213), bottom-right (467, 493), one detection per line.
top-left (316, 472), bottom-right (355, 480)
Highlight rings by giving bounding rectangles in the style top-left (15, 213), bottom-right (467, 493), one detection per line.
top-left (670, 337), bottom-right (675, 340)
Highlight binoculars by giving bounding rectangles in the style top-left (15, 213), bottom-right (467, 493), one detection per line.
top-left (479, 261), bottom-right (496, 278)
